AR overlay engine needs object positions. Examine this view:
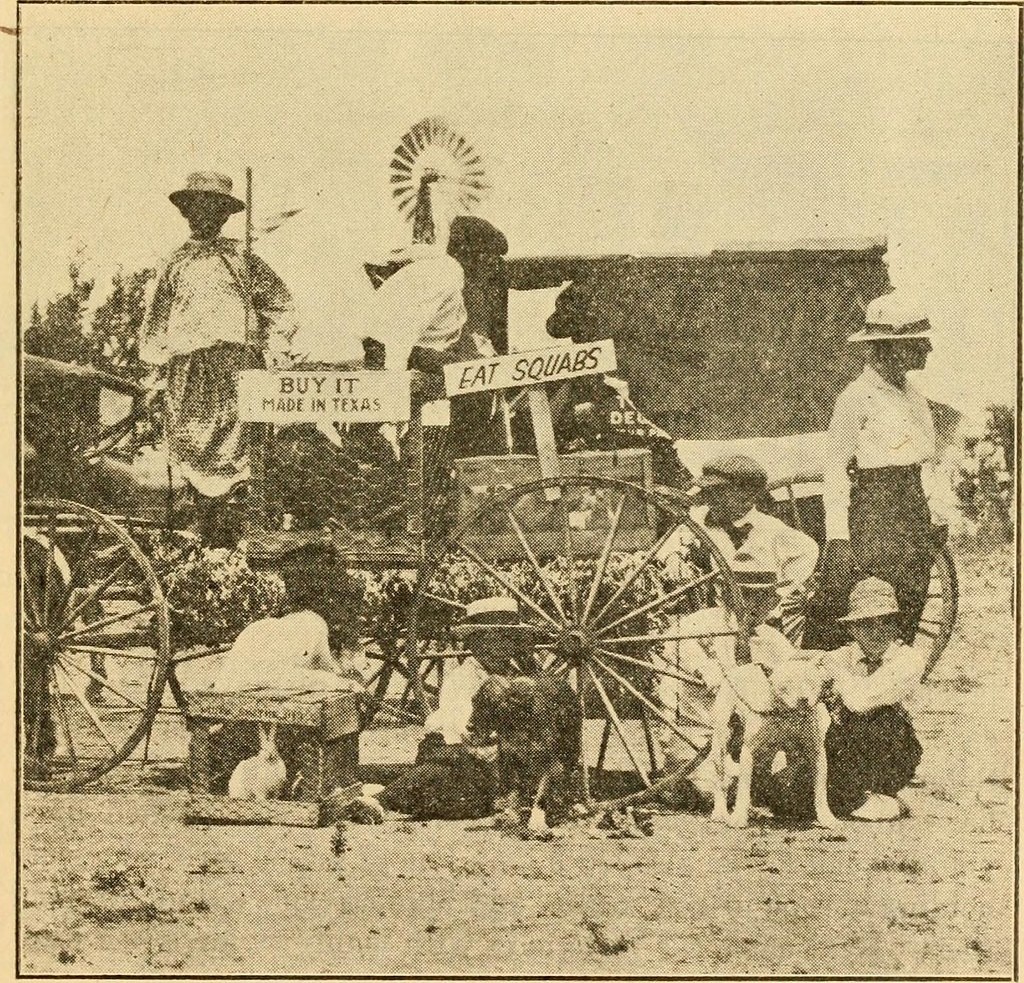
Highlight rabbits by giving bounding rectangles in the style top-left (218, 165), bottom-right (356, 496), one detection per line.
top-left (227, 722), bottom-right (288, 801)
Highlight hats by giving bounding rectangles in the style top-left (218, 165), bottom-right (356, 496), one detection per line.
top-left (834, 576), bottom-right (900, 625)
top-left (846, 293), bottom-right (939, 341)
top-left (168, 170), bottom-right (245, 214)
top-left (691, 456), bottom-right (768, 488)
top-left (715, 558), bottom-right (793, 588)
top-left (447, 596), bottom-right (538, 640)
top-left (449, 214), bottom-right (510, 256)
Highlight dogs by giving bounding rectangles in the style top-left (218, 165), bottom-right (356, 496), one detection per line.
top-left (708, 644), bottom-right (842, 829)
top-left (462, 672), bottom-right (584, 842)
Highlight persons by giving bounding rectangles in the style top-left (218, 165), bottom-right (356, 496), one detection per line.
top-left (346, 596), bottom-right (542, 826)
top-left (817, 290), bottom-right (941, 647)
top-left (630, 557), bottom-right (827, 814)
top-left (136, 171), bottom-right (294, 527)
top-left (270, 539), bottom-right (369, 683)
top-left (655, 455), bottom-right (819, 635)
top-left (818, 577), bottom-right (924, 822)
top-left (361, 216), bottom-right (507, 456)
